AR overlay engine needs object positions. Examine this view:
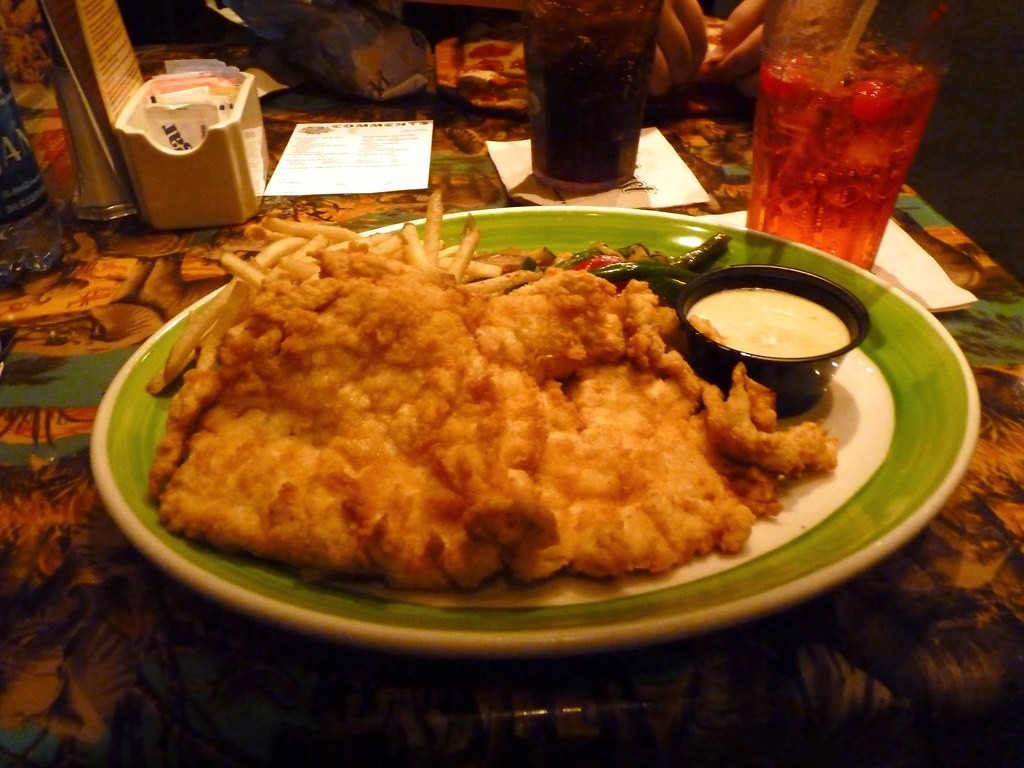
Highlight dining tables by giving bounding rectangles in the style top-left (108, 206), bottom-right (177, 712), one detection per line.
top-left (0, 43), bottom-right (1024, 768)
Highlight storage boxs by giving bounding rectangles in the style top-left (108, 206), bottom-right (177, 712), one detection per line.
top-left (112, 70), bottom-right (269, 230)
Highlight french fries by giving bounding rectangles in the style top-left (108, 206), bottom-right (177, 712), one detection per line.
top-left (146, 191), bottom-right (555, 397)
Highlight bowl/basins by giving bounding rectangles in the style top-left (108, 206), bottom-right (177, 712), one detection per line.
top-left (675, 263), bottom-right (870, 419)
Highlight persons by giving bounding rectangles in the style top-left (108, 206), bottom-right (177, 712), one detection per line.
top-left (646, 0), bottom-right (766, 104)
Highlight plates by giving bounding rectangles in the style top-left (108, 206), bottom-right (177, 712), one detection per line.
top-left (88, 203), bottom-right (981, 653)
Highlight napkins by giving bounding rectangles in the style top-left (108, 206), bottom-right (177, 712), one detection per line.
top-left (484, 126), bottom-right (711, 210)
top-left (696, 210), bottom-right (980, 314)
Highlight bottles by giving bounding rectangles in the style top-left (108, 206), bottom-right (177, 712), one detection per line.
top-left (0, 62), bottom-right (65, 289)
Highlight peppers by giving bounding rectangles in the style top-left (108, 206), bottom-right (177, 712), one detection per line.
top-left (557, 233), bottom-right (731, 284)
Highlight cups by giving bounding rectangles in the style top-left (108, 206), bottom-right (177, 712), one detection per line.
top-left (521, 0), bottom-right (665, 193)
top-left (742, 0), bottom-right (963, 270)
